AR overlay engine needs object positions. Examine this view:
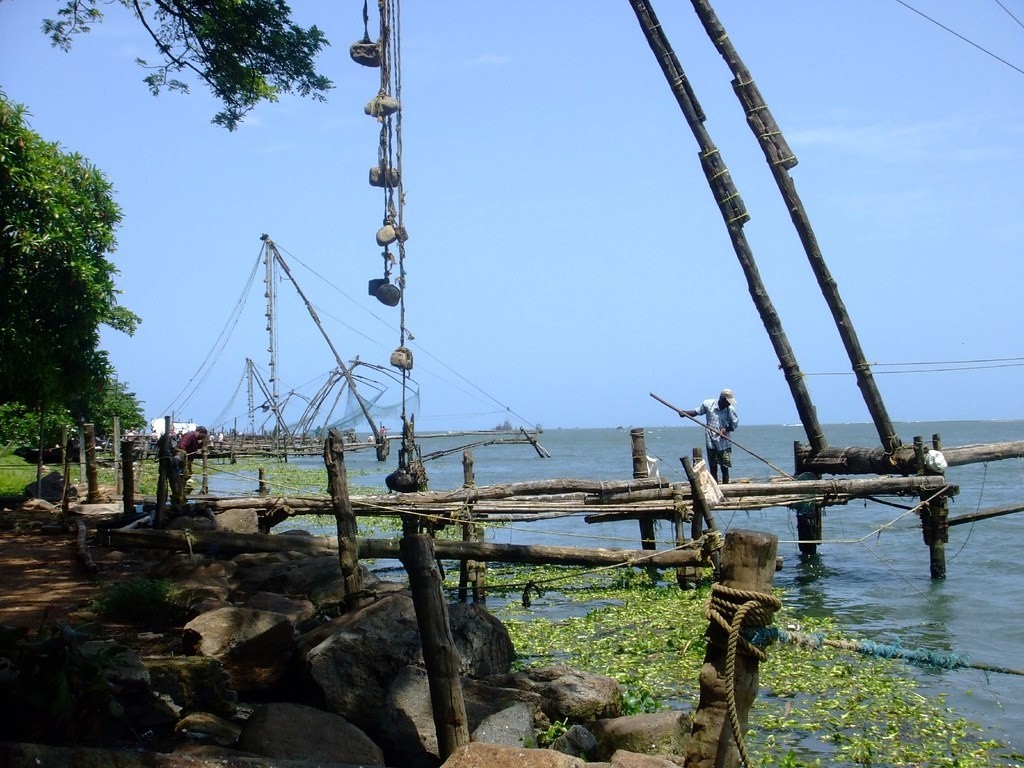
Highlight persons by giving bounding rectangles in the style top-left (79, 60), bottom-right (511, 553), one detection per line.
top-left (150, 430), bottom-right (158, 450)
top-left (208, 431), bottom-right (224, 452)
top-left (121, 426), bottom-right (139, 441)
top-left (381, 425), bottom-right (390, 435)
top-left (680, 389), bottom-right (738, 482)
top-left (160, 425), bottom-right (208, 503)
top-left (367, 434), bottom-right (374, 442)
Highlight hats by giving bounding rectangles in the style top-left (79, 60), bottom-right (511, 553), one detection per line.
top-left (721, 390), bottom-right (736, 405)
